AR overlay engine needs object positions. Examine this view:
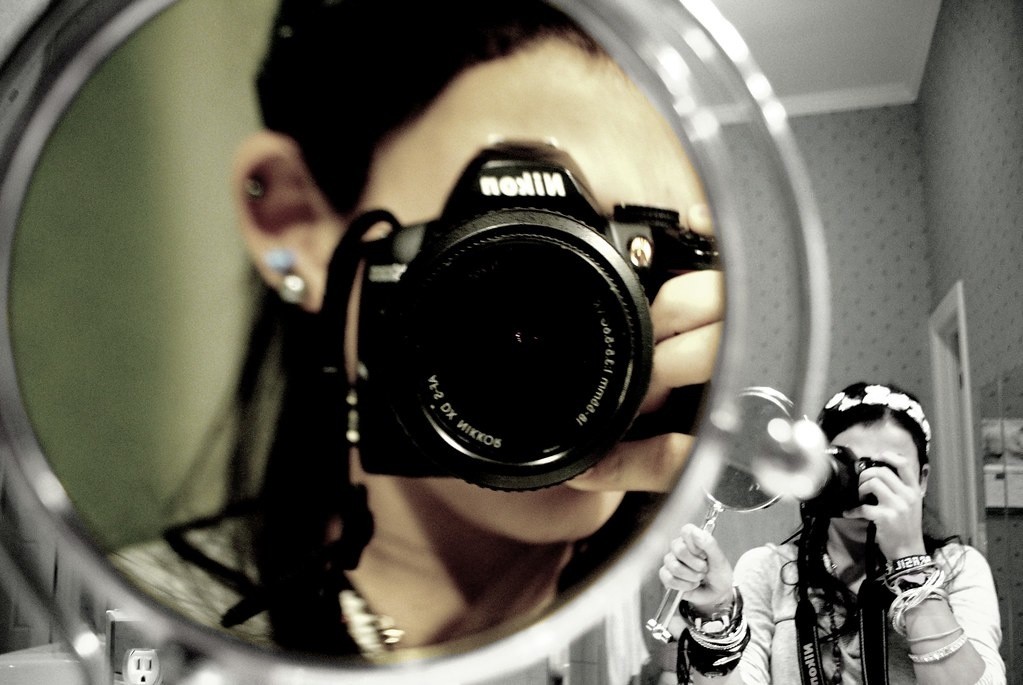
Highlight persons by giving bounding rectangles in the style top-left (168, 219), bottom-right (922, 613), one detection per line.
top-left (657, 382), bottom-right (1010, 685)
top-left (104, 1), bottom-right (727, 656)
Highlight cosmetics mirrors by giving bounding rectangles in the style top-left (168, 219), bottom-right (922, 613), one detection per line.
top-left (0, 0), bottom-right (831, 683)
top-left (648, 386), bottom-right (794, 646)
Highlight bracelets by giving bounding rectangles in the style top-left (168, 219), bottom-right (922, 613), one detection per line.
top-left (885, 552), bottom-right (967, 664)
top-left (678, 587), bottom-right (750, 684)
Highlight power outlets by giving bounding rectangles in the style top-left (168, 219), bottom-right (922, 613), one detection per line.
top-left (121, 646), bottom-right (164, 685)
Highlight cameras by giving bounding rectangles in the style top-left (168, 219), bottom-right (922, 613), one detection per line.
top-left (353, 139), bottom-right (723, 493)
top-left (799, 444), bottom-right (901, 519)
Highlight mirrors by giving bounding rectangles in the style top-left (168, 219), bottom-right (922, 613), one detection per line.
top-left (6, 3), bottom-right (727, 671)
